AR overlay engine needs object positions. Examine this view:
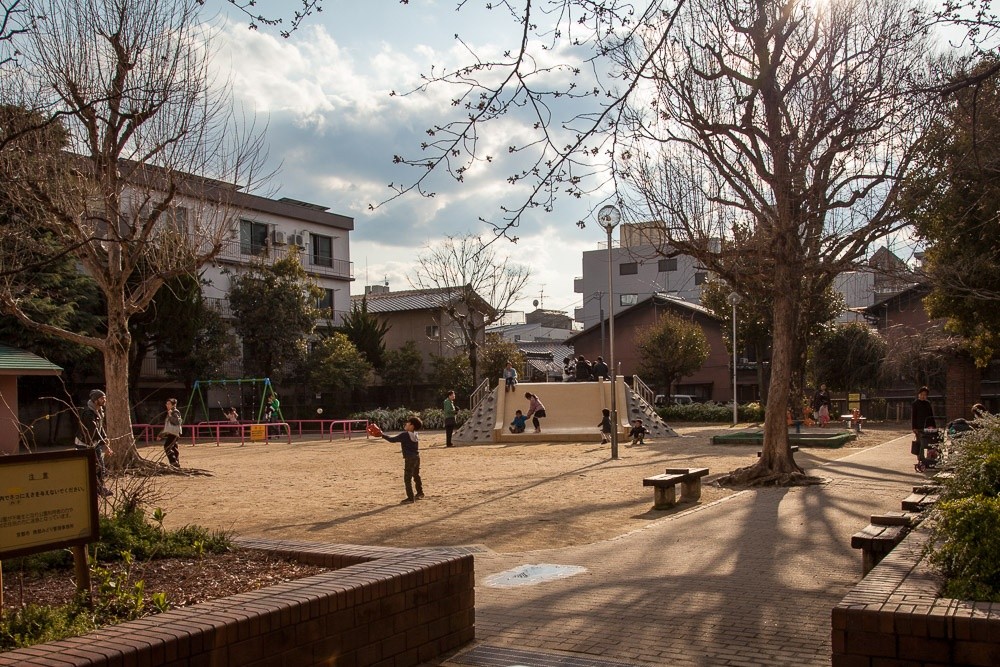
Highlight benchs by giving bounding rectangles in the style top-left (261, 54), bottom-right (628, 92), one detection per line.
top-left (851, 469), bottom-right (957, 577)
top-left (643, 467), bottom-right (709, 510)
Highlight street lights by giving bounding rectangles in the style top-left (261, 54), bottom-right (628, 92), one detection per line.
top-left (598, 205), bottom-right (622, 457)
top-left (727, 291), bottom-right (742, 424)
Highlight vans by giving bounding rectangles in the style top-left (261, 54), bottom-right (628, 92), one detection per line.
top-left (655, 394), bottom-right (701, 406)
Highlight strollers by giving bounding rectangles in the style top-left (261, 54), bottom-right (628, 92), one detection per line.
top-left (914, 415), bottom-right (948, 472)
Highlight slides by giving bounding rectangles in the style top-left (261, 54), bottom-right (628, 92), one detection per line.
top-left (491, 374), bottom-right (632, 444)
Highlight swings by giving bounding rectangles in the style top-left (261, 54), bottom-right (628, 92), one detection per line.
top-left (208, 383), bottom-right (236, 425)
top-left (238, 382), bottom-right (257, 428)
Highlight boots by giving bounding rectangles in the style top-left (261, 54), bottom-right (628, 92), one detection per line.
top-left (534, 426), bottom-right (541, 433)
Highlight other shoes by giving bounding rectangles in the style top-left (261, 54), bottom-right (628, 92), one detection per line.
top-left (601, 439), bottom-right (607, 444)
top-left (415, 493), bottom-right (425, 500)
top-left (505, 385), bottom-right (509, 392)
top-left (400, 497), bottom-right (414, 504)
top-left (509, 427), bottom-right (515, 433)
top-left (512, 385), bottom-right (515, 392)
top-left (514, 427), bottom-right (518, 434)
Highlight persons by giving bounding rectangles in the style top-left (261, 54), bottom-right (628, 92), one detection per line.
top-left (972, 404), bottom-right (986, 419)
top-left (509, 410), bottom-right (530, 434)
top-left (224, 407), bottom-right (238, 433)
top-left (156, 399), bottom-right (182, 468)
top-left (813, 383), bottom-right (831, 427)
top-left (367, 418), bottom-right (425, 504)
top-left (912, 388), bottom-right (936, 467)
top-left (503, 363), bottom-right (518, 392)
top-left (597, 409), bottom-right (611, 444)
top-left (563, 354), bottom-right (608, 382)
top-left (81, 389), bottom-right (113, 496)
top-left (265, 393), bottom-right (280, 438)
top-left (629, 420), bottom-right (647, 444)
top-left (444, 390), bottom-right (458, 447)
top-left (525, 392), bottom-right (546, 432)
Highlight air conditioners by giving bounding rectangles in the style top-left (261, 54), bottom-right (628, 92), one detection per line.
top-left (271, 231), bottom-right (287, 244)
top-left (291, 234), bottom-right (305, 247)
